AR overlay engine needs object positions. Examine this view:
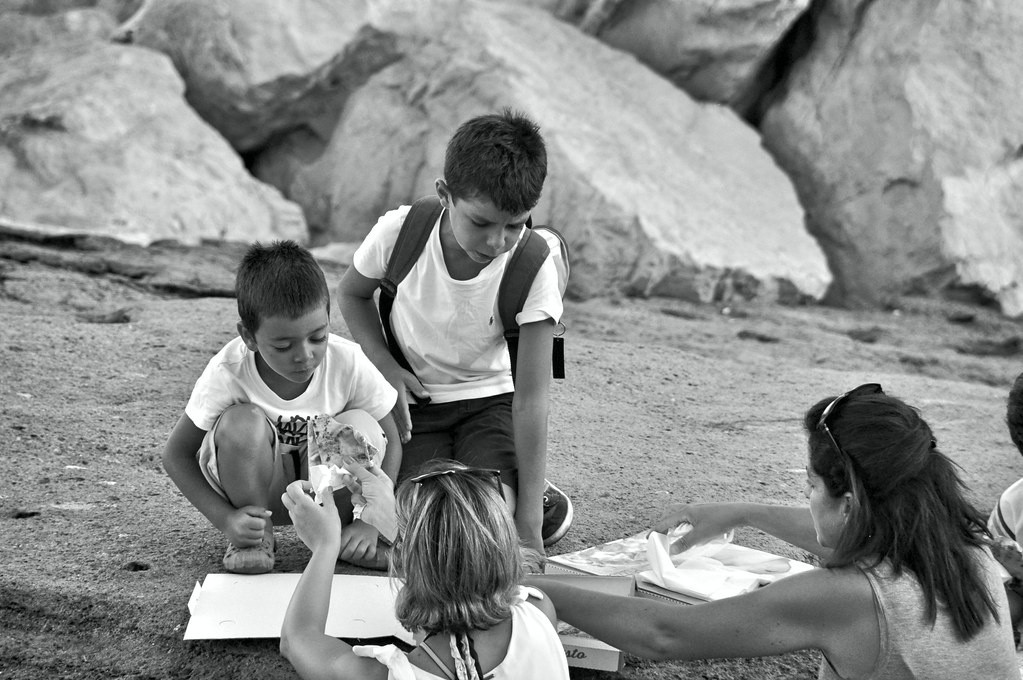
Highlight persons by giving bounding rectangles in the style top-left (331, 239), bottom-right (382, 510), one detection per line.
top-left (281, 458), bottom-right (572, 680)
top-left (161, 239), bottom-right (404, 573)
top-left (522, 382), bottom-right (1023, 680)
top-left (335, 112), bottom-right (573, 545)
top-left (982, 372), bottom-right (1023, 652)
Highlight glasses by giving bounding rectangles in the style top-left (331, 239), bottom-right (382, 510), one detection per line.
top-left (410, 468), bottom-right (504, 513)
top-left (816, 382), bottom-right (888, 469)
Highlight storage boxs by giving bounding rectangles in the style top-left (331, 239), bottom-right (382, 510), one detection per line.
top-left (183, 573), bottom-right (626, 673)
top-left (542, 531), bottom-right (816, 609)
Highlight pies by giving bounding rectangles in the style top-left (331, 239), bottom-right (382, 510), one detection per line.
top-left (307, 420), bottom-right (322, 493)
top-left (312, 413), bottom-right (378, 470)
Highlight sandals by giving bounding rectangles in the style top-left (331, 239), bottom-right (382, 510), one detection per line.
top-left (338, 538), bottom-right (398, 570)
top-left (222, 531), bottom-right (274, 574)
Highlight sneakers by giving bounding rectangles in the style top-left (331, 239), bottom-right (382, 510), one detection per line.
top-left (541, 479), bottom-right (573, 545)
